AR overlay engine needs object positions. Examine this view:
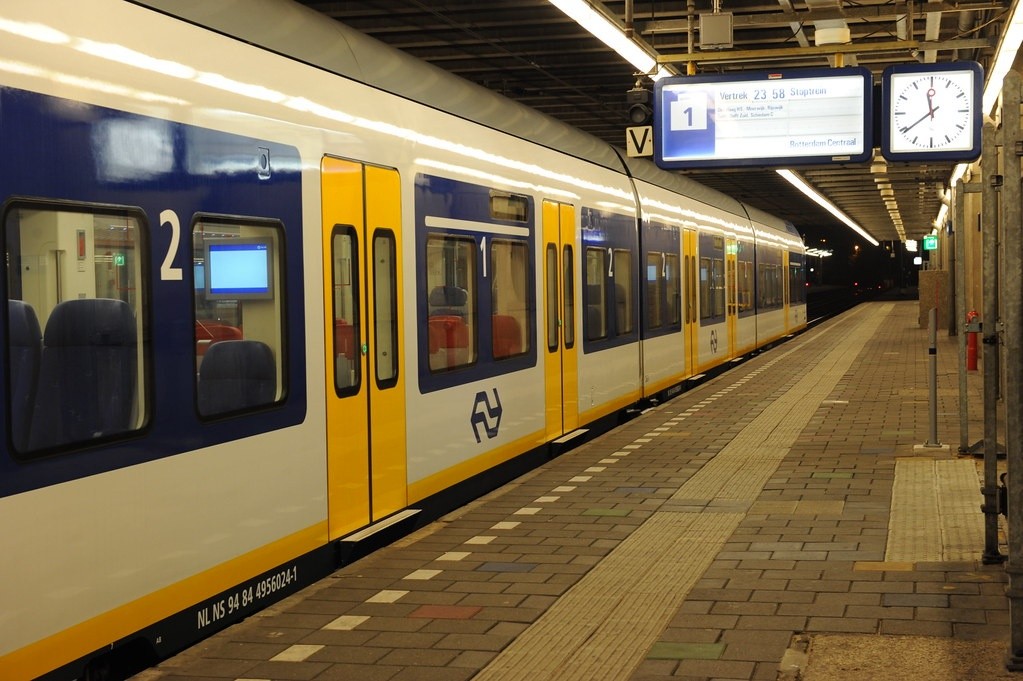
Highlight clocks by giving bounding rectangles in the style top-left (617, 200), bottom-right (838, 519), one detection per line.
top-left (878, 64), bottom-right (980, 166)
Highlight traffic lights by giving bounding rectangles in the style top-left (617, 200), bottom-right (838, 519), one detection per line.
top-left (625, 89), bottom-right (654, 127)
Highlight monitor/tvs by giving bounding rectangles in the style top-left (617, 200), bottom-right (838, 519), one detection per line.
top-left (701, 268), bottom-right (707, 281)
top-left (204, 236), bottom-right (275, 300)
top-left (647, 264), bottom-right (656, 281)
top-left (194, 263), bottom-right (205, 292)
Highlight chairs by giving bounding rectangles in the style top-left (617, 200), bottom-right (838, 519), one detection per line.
top-left (0, 270), bottom-right (628, 468)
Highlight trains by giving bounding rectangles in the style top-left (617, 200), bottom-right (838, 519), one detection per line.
top-left (0, 0), bottom-right (808, 680)
